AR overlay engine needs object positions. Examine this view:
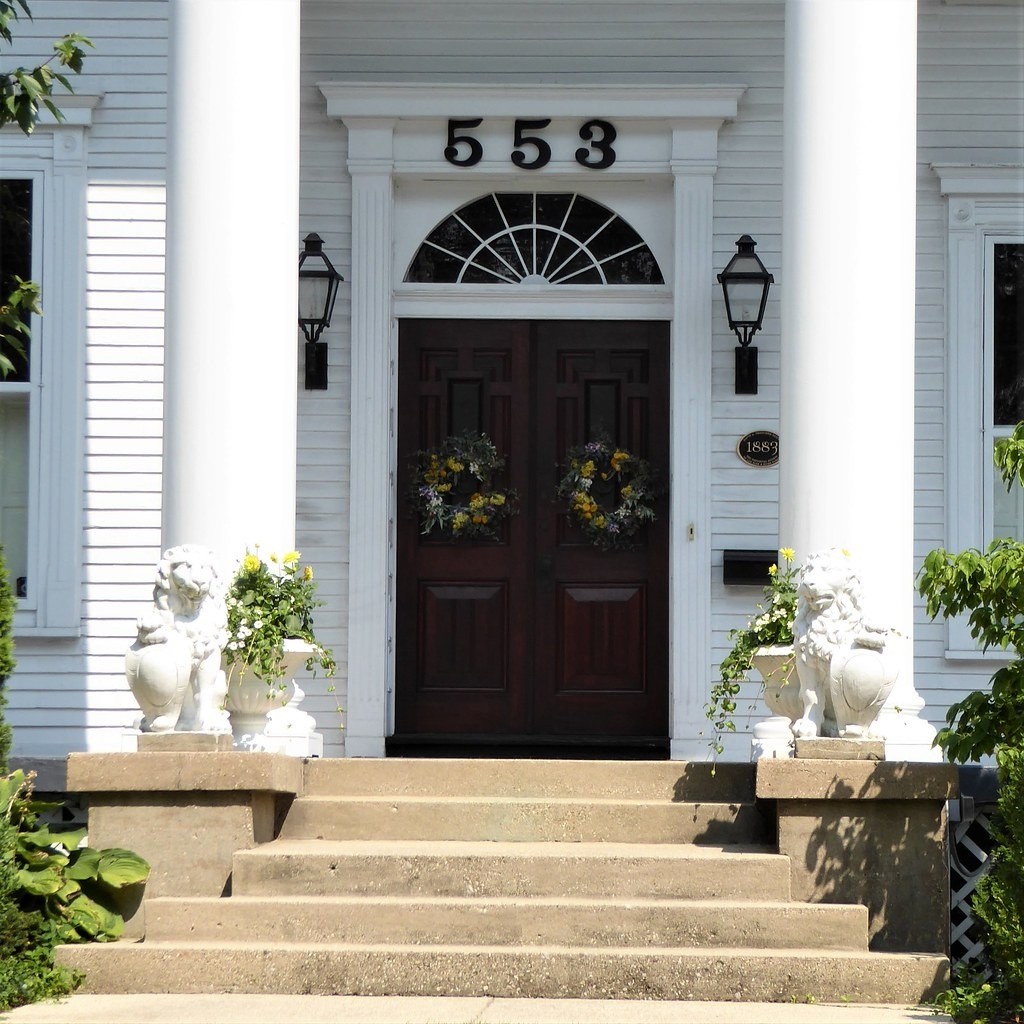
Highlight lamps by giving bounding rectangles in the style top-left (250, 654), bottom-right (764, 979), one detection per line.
top-left (299, 232), bottom-right (344, 391)
top-left (717, 233), bottom-right (774, 395)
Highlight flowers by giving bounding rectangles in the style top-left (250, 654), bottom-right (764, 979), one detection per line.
top-left (551, 430), bottom-right (658, 555)
top-left (403, 428), bottom-right (521, 543)
top-left (698, 547), bottom-right (852, 779)
top-left (221, 542), bottom-right (345, 732)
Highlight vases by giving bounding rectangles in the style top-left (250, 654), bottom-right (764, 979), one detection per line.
top-left (748, 645), bottom-right (804, 740)
top-left (220, 640), bottom-right (321, 736)
